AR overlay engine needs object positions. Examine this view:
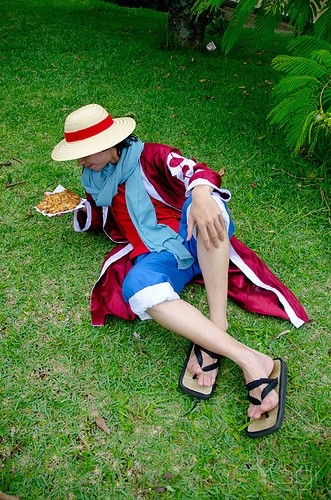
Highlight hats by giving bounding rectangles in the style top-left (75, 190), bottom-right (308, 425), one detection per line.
top-left (51, 104), bottom-right (136, 162)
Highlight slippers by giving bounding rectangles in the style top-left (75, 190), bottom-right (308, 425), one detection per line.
top-left (178, 342), bottom-right (221, 400)
top-left (246, 358), bottom-right (287, 438)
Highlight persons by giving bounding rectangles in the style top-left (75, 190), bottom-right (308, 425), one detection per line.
top-left (43, 103), bottom-right (310, 438)
